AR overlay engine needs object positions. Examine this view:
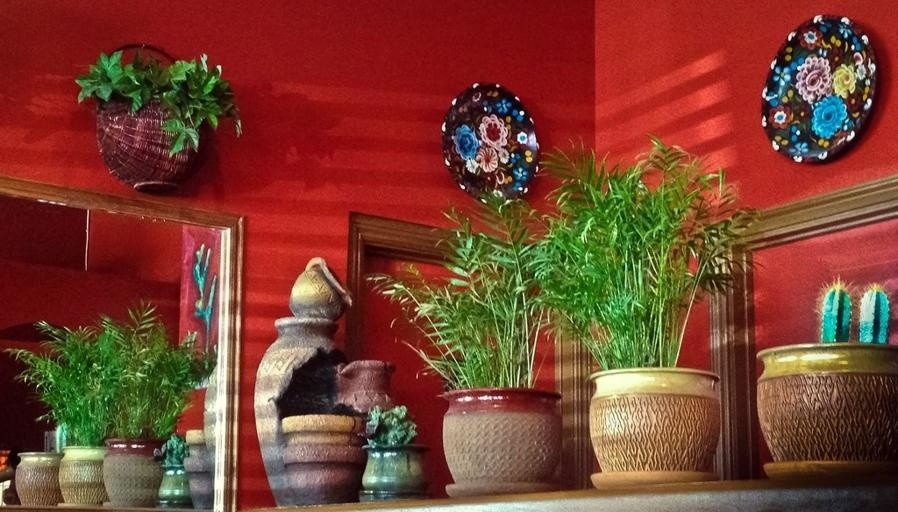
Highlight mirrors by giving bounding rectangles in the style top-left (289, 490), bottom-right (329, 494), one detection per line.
top-left (0, 177), bottom-right (244, 510)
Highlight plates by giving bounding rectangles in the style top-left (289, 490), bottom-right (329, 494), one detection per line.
top-left (760, 14), bottom-right (880, 163)
top-left (442, 82), bottom-right (540, 206)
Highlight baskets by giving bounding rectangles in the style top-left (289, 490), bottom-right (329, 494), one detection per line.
top-left (96, 44), bottom-right (203, 192)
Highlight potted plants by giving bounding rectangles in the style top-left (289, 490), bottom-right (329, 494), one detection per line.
top-left (75, 50), bottom-right (245, 189)
top-left (1, 299), bottom-right (216, 508)
top-left (367, 182), bottom-right (565, 499)
top-left (521, 137), bottom-right (762, 492)
top-left (754, 274), bottom-right (898, 487)
top-left (363, 404), bottom-right (428, 500)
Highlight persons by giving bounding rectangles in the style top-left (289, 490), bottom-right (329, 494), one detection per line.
top-left (1, 447), bottom-right (24, 505)
